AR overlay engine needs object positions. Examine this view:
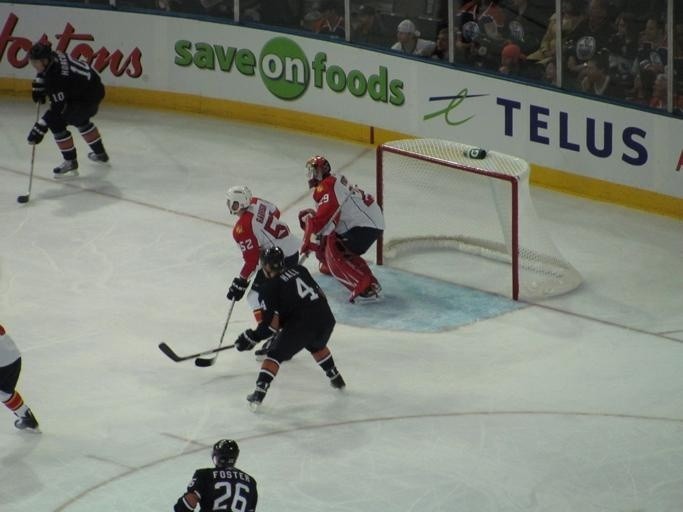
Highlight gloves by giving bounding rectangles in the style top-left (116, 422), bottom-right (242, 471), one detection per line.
top-left (234, 328), bottom-right (260, 351)
top-left (31, 79), bottom-right (47, 105)
top-left (227, 277), bottom-right (250, 301)
top-left (28, 121), bottom-right (48, 144)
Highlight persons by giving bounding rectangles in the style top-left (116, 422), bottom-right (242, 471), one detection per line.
top-left (112, 0), bottom-right (683, 113)
top-left (173, 439), bottom-right (258, 512)
top-left (224, 186), bottom-right (301, 361)
top-left (297, 154), bottom-right (386, 302)
top-left (236, 246), bottom-right (347, 413)
top-left (0, 323), bottom-right (42, 432)
top-left (26, 43), bottom-right (109, 182)
top-left (296, 209), bottom-right (332, 275)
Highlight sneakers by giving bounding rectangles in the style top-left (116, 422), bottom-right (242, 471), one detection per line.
top-left (247, 394), bottom-right (264, 403)
top-left (331, 374), bottom-right (346, 389)
top-left (360, 280), bottom-right (382, 298)
top-left (88, 152), bottom-right (109, 162)
top-left (54, 159), bottom-right (78, 173)
top-left (15, 408), bottom-right (39, 429)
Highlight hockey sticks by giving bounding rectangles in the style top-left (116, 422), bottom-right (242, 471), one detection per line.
top-left (195, 296), bottom-right (235, 366)
top-left (17, 101), bottom-right (39, 203)
top-left (158, 343), bottom-right (238, 361)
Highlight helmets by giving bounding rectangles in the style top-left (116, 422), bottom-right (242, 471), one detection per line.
top-left (258, 246), bottom-right (284, 269)
top-left (305, 156), bottom-right (332, 189)
top-left (27, 42), bottom-right (51, 60)
top-left (225, 185), bottom-right (253, 215)
top-left (211, 439), bottom-right (240, 467)
